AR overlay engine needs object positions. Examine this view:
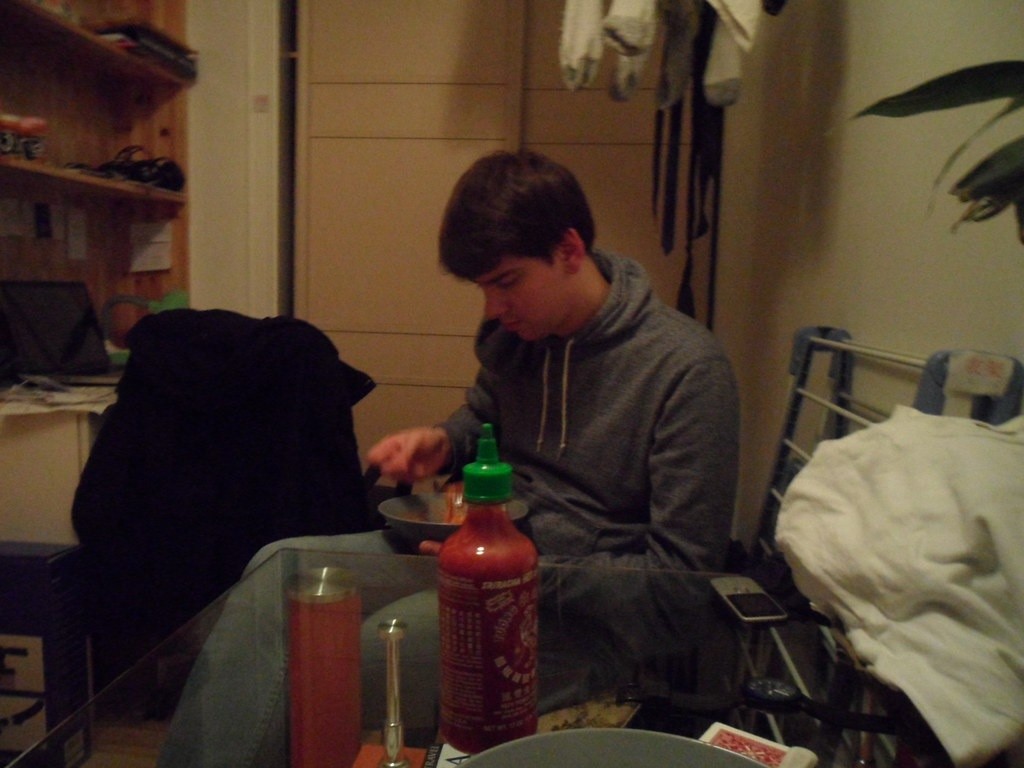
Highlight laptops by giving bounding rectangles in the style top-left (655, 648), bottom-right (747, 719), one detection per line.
top-left (0, 280), bottom-right (125, 385)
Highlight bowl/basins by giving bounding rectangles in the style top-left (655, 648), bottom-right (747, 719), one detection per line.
top-left (377, 491), bottom-right (528, 543)
top-left (452, 728), bottom-right (767, 768)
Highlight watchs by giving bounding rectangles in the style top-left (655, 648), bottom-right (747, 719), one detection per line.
top-left (616, 676), bottom-right (896, 735)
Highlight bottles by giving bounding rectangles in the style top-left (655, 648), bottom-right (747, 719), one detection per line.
top-left (438, 423), bottom-right (537, 755)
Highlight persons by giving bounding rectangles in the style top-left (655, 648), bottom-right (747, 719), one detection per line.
top-left (158, 149), bottom-right (741, 768)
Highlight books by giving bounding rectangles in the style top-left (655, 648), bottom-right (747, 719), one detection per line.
top-left (86, 17), bottom-right (199, 80)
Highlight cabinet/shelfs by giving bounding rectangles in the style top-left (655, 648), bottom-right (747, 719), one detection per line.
top-left (1, 1), bottom-right (195, 203)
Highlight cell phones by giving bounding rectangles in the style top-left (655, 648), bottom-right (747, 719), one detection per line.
top-left (709, 576), bottom-right (787, 625)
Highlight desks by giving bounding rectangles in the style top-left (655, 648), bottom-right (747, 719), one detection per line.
top-left (7, 546), bottom-right (958, 766)
